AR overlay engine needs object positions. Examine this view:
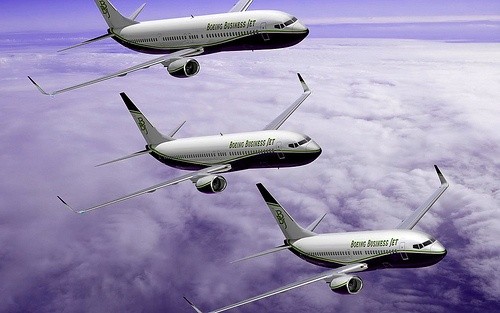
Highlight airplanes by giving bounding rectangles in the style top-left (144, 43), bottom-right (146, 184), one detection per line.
top-left (56, 72), bottom-right (322, 215)
top-left (183, 165), bottom-right (449, 313)
top-left (28, 0), bottom-right (310, 97)
